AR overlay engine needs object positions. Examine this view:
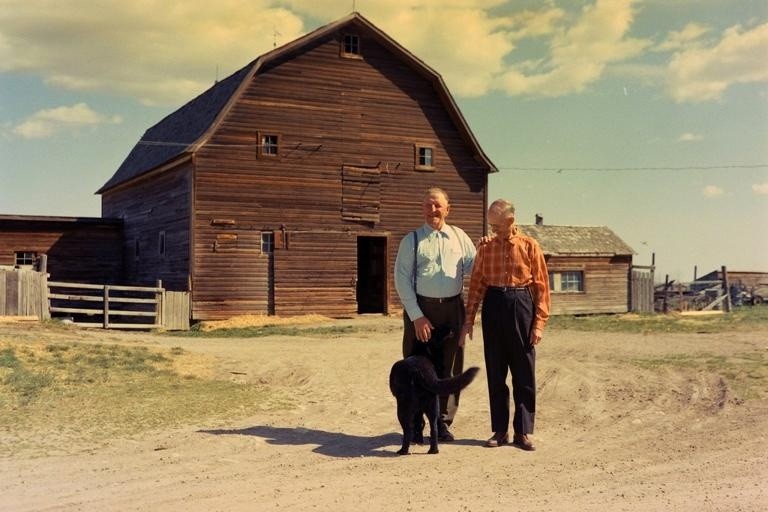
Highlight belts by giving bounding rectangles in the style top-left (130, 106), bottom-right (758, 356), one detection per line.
top-left (487, 284), bottom-right (529, 293)
top-left (415, 293), bottom-right (464, 304)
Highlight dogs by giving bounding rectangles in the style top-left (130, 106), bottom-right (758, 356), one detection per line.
top-left (388, 353), bottom-right (480, 456)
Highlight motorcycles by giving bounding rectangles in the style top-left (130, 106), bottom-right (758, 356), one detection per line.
top-left (731, 285), bottom-right (763, 306)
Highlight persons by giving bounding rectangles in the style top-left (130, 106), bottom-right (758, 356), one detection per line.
top-left (392, 184), bottom-right (495, 445)
top-left (457, 198), bottom-right (551, 452)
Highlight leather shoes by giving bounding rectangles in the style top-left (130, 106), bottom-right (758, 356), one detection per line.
top-left (513, 432), bottom-right (536, 451)
top-left (486, 431), bottom-right (509, 447)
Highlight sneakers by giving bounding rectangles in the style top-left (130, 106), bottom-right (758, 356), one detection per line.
top-left (411, 425), bottom-right (424, 444)
top-left (430, 423), bottom-right (454, 441)
